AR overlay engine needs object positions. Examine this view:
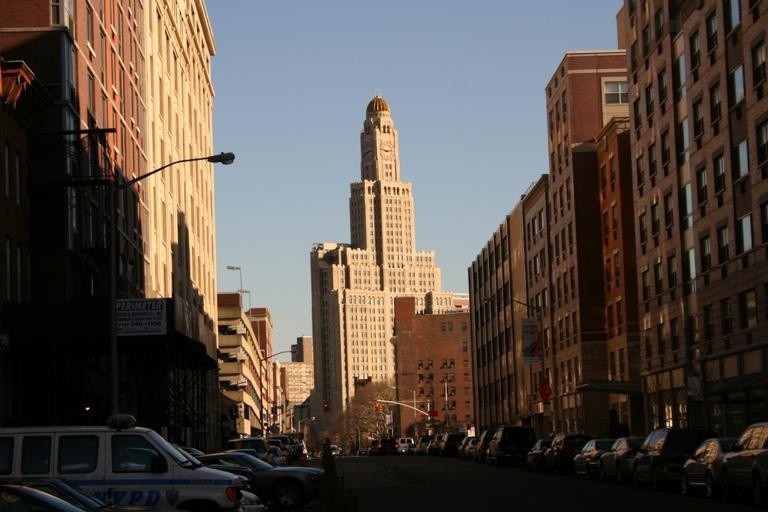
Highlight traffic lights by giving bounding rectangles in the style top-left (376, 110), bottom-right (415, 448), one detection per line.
top-left (373, 402), bottom-right (379, 413)
top-left (379, 404), bottom-right (383, 413)
top-left (323, 400), bottom-right (329, 412)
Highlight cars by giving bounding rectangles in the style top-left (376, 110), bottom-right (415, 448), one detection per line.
top-left (681, 435), bottom-right (739, 501)
top-left (0, 477), bottom-right (118, 510)
top-left (526, 437), bottom-right (556, 471)
top-left (351, 429), bottom-right (497, 462)
top-left (572, 438), bottom-right (617, 480)
top-left (180, 430), bottom-right (343, 511)
top-left (721, 420), bottom-right (767, 503)
top-left (599, 433), bottom-right (648, 485)
top-left (59, 446), bottom-right (160, 473)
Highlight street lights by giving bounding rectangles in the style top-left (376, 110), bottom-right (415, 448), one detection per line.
top-left (258, 347), bottom-right (301, 432)
top-left (226, 265), bottom-right (243, 310)
top-left (482, 297), bottom-right (550, 438)
top-left (247, 316), bottom-right (260, 344)
top-left (391, 386), bottom-right (417, 429)
top-left (238, 288), bottom-right (252, 326)
top-left (297, 415), bottom-right (316, 433)
top-left (105, 149), bottom-right (235, 425)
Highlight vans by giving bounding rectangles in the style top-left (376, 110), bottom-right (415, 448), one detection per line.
top-left (0, 411), bottom-right (244, 512)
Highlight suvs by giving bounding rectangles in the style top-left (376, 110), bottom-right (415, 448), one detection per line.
top-left (631, 427), bottom-right (719, 490)
top-left (541, 431), bottom-right (597, 474)
top-left (486, 424), bottom-right (538, 470)
